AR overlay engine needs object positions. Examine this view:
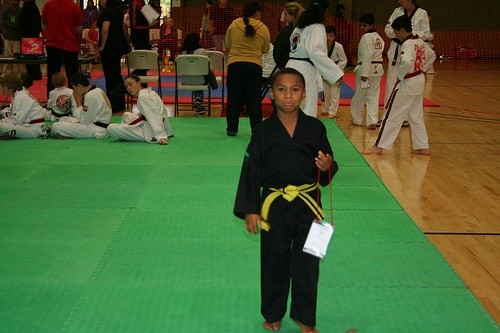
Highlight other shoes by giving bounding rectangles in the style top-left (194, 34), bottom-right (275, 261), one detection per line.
top-left (402, 120), bottom-right (412, 127)
top-left (226, 129), bottom-right (238, 136)
top-left (376, 118), bottom-right (384, 127)
top-left (193, 96), bottom-right (202, 108)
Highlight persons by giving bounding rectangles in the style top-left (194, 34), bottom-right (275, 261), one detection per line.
top-left (224, 0), bottom-right (278, 136)
top-left (97, 0), bottom-right (126, 113)
top-left (2, 0), bottom-right (22, 58)
top-left (41, 0), bottom-right (84, 108)
top-left (179, 32), bottom-right (218, 108)
top-left (157, 17), bottom-right (178, 65)
top-left (350, 13), bottom-right (385, 130)
top-left (200, 1), bottom-right (214, 50)
top-left (83, 0), bottom-right (97, 28)
top-left (422, 13), bottom-right (440, 107)
top-left (321, 25), bottom-right (348, 118)
top-left (285, 0), bottom-right (345, 118)
top-left (273, 1), bottom-right (306, 69)
top-left (15, 0), bottom-right (42, 57)
top-left (375, 0), bottom-right (430, 127)
top-left (85, 20), bottom-right (100, 64)
top-left (0, 71), bottom-right (174, 145)
top-left (130, 16), bottom-right (152, 50)
top-left (360, 16), bottom-right (437, 155)
top-left (233, 68), bottom-right (338, 333)
top-left (210, 0), bottom-right (237, 52)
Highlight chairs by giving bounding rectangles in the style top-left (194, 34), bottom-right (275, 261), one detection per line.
top-left (205, 51), bottom-right (225, 109)
top-left (174, 55), bottom-right (212, 117)
top-left (127, 50), bottom-right (162, 109)
top-left (261, 64), bottom-right (277, 112)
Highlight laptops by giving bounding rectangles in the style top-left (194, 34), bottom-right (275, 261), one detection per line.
top-left (16, 37), bottom-right (45, 58)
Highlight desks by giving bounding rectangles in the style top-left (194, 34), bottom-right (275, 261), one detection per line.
top-left (0, 55), bottom-right (95, 72)
